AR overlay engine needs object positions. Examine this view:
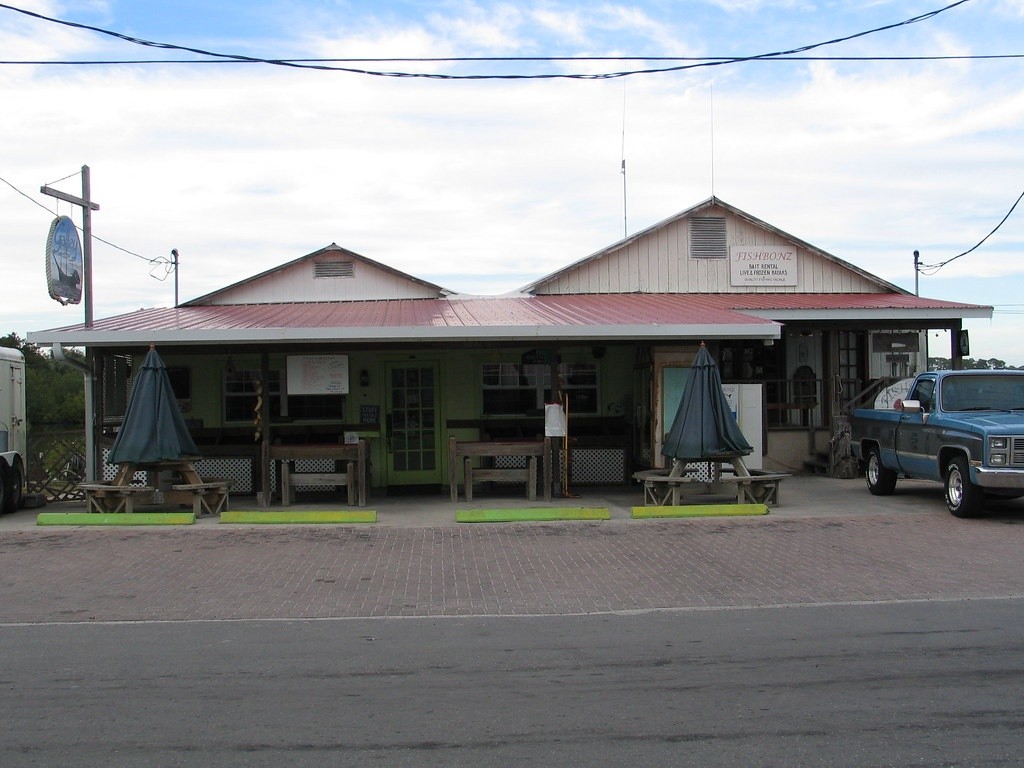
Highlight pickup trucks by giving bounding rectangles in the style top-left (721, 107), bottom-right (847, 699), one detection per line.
top-left (853, 369), bottom-right (1024, 516)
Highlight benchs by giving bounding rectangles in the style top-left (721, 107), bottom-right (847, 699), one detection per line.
top-left (80, 478), bottom-right (233, 517)
top-left (630, 465), bottom-right (793, 507)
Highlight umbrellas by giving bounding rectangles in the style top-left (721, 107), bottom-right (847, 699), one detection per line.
top-left (106, 343), bottom-right (203, 464)
top-left (660, 341), bottom-right (753, 457)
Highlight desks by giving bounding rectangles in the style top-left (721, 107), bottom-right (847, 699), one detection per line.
top-left (663, 448), bottom-right (756, 484)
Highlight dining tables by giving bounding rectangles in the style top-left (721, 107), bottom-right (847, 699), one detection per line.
top-left (108, 451), bottom-right (211, 490)
